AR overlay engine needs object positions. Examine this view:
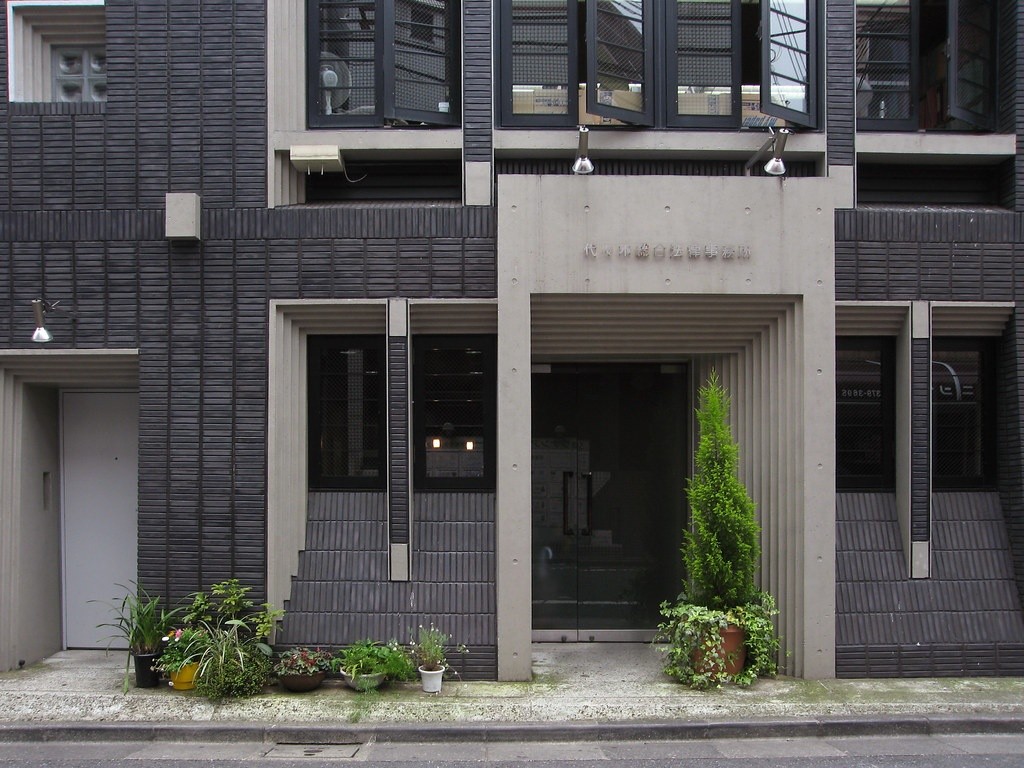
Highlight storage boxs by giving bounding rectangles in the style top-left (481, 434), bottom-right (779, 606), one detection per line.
top-left (677, 93), bottom-right (787, 127)
top-left (512, 90), bottom-right (643, 125)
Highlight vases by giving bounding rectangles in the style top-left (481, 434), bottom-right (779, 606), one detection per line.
top-left (170, 661), bottom-right (199, 690)
top-left (418, 665), bottom-right (446, 692)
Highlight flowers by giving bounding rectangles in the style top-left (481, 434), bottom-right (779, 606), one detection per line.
top-left (408, 623), bottom-right (468, 693)
top-left (150, 628), bottom-right (211, 686)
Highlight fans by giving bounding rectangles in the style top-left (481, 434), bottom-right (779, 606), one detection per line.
top-left (321, 50), bottom-right (352, 115)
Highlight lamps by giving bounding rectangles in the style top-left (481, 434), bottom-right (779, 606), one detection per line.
top-left (744, 127), bottom-right (789, 176)
top-left (573, 124), bottom-right (594, 174)
top-left (32, 297), bottom-right (58, 342)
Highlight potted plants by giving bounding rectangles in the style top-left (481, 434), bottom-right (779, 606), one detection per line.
top-left (332, 639), bottom-right (417, 722)
top-left (85, 580), bottom-right (190, 689)
top-left (649, 367), bottom-right (793, 692)
top-left (273, 648), bottom-right (337, 693)
